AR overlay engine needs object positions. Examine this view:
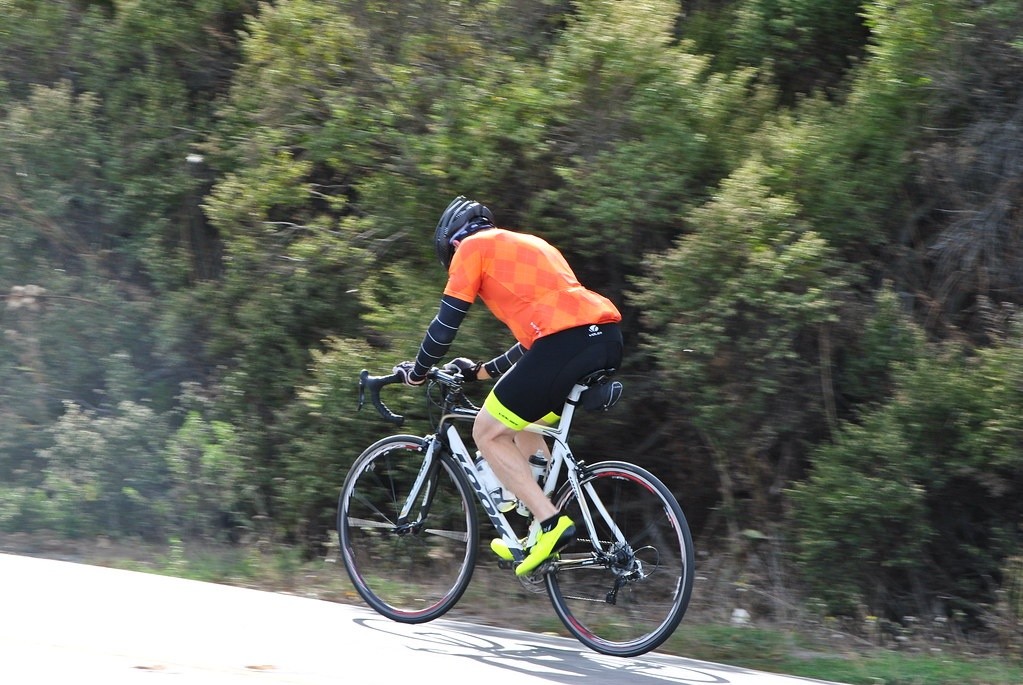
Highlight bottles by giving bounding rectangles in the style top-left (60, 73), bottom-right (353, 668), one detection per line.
top-left (474, 451), bottom-right (517, 513)
top-left (516, 449), bottom-right (547, 518)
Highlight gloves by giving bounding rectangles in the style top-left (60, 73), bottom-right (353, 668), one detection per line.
top-left (443, 357), bottom-right (482, 382)
top-left (393, 360), bottom-right (427, 387)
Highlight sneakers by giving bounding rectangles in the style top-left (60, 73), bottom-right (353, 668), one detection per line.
top-left (490, 537), bottom-right (527, 561)
top-left (515, 515), bottom-right (576, 576)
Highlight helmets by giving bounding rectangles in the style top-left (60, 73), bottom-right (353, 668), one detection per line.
top-left (433, 194), bottom-right (495, 270)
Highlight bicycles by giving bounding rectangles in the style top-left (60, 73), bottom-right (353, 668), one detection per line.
top-left (337, 356), bottom-right (696, 659)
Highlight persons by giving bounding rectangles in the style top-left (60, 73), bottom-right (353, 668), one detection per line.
top-left (391, 195), bottom-right (622, 577)
